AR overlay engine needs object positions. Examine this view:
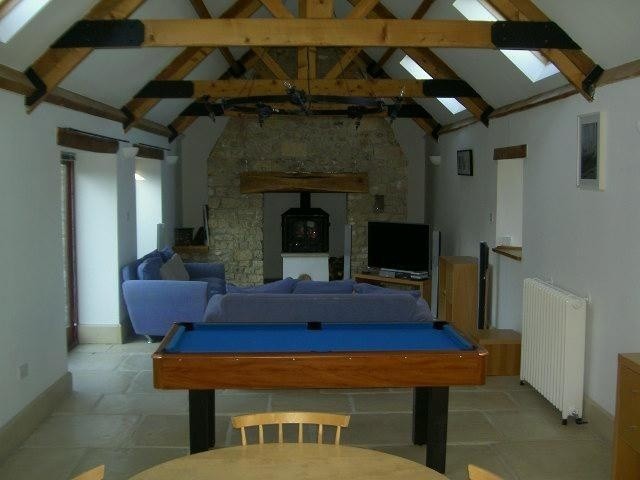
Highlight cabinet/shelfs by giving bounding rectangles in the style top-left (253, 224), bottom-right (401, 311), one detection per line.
top-left (611, 352), bottom-right (640, 480)
top-left (355, 272), bottom-right (433, 306)
top-left (439, 253), bottom-right (521, 376)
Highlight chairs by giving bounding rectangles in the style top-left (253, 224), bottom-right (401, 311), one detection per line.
top-left (70, 412), bottom-right (504, 479)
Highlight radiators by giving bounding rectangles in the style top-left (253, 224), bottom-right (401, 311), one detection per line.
top-left (520, 277), bottom-right (586, 425)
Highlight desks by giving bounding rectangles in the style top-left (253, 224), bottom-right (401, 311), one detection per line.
top-left (152, 322), bottom-right (487, 472)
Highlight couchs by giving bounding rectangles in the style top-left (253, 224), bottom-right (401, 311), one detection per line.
top-left (120, 248), bottom-right (228, 340)
top-left (204, 278), bottom-right (432, 323)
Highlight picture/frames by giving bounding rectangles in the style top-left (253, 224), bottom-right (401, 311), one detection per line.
top-left (456, 149), bottom-right (473, 176)
top-left (576, 112), bottom-right (605, 191)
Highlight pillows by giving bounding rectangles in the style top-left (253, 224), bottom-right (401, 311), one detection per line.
top-left (227, 277), bottom-right (294, 294)
top-left (293, 278), bottom-right (354, 293)
top-left (159, 253), bottom-right (191, 280)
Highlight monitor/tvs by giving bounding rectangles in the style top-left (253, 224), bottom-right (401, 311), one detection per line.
top-left (367, 222), bottom-right (431, 275)
